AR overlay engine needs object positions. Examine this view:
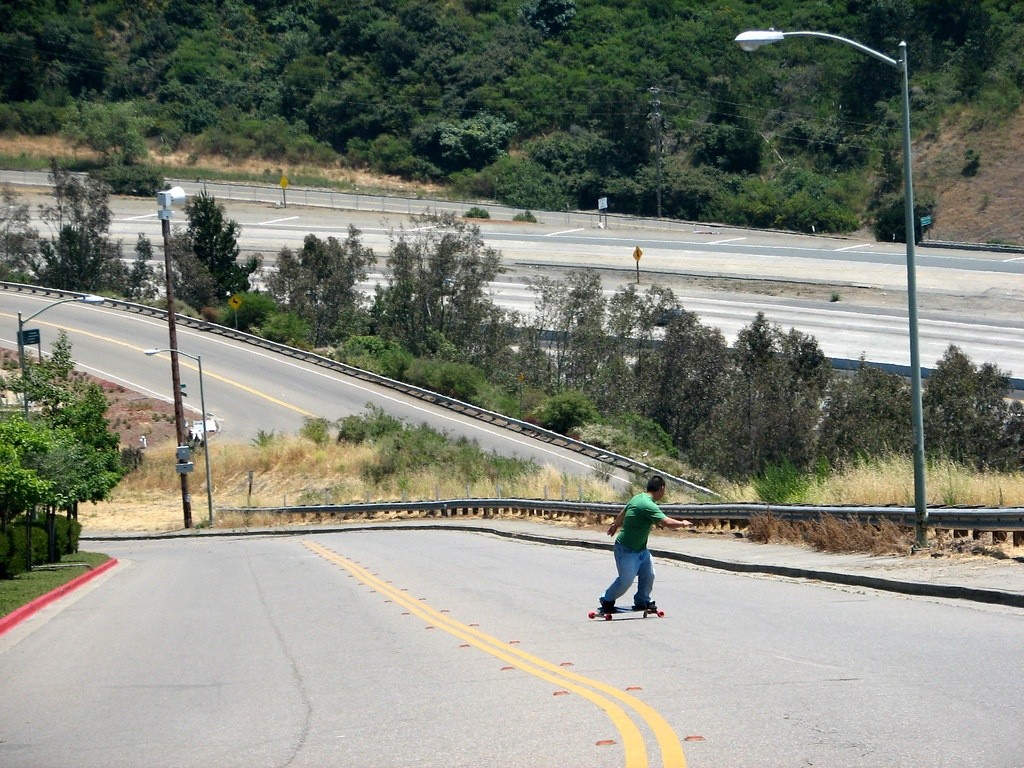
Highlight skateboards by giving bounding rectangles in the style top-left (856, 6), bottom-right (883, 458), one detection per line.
top-left (587, 600), bottom-right (665, 621)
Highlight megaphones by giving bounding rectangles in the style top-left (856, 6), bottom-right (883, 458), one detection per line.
top-left (166, 186), bottom-right (186, 206)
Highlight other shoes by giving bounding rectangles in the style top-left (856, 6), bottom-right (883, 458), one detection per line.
top-left (634, 598), bottom-right (657, 610)
top-left (599, 596), bottom-right (615, 615)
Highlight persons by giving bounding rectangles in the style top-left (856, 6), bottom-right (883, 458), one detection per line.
top-left (600, 475), bottom-right (692, 610)
top-left (189, 429), bottom-right (204, 447)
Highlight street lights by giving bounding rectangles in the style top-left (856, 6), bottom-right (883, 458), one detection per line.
top-left (735, 28), bottom-right (930, 549)
top-left (18, 295), bottom-right (106, 421)
top-left (142, 347), bottom-right (213, 528)
